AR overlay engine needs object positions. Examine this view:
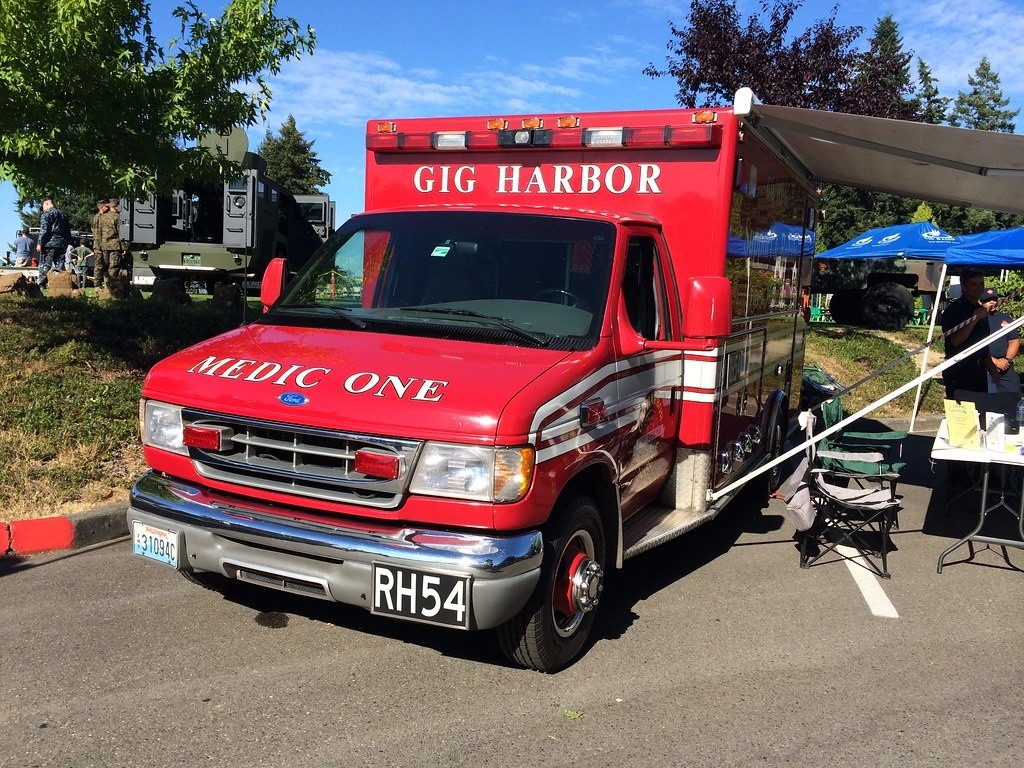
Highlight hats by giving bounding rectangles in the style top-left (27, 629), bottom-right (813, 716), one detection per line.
top-left (23, 229), bottom-right (30, 233)
top-left (109, 199), bottom-right (119, 205)
top-left (97, 198), bottom-right (106, 206)
top-left (81, 239), bottom-right (86, 244)
top-left (980, 289), bottom-right (998, 303)
top-left (40, 197), bottom-right (51, 211)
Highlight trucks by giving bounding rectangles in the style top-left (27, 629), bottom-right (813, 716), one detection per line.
top-left (115, 126), bottom-right (336, 304)
top-left (121, 88), bottom-right (1023, 674)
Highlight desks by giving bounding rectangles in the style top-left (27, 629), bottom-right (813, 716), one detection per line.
top-left (931, 418), bottom-right (1024, 574)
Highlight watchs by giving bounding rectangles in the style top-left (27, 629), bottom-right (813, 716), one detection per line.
top-left (1005, 357), bottom-right (1013, 364)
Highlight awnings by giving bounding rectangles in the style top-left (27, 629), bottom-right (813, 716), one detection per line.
top-left (733, 87), bottom-right (1024, 217)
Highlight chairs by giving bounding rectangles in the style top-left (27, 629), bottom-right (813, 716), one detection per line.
top-left (817, 397), bottom-right (908, 500)
top-left (799, 408), bottom-right (901, 579)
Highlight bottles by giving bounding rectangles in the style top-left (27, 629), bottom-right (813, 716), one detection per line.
top-left (1016, 398), bottom-right (1024, 433)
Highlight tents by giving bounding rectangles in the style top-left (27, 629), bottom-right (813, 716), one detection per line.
top-left (813, 221), bottom-right (1024, 437)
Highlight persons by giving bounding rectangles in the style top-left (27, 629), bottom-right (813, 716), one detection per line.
top-left (92, 197), bottom-right (127, 290)
top-left (11, 229), bottom-right (32, 269)
top-left (977, 288), bottom-right (1021, 393)
top-left (71, 240), bottom-right (92, 289)
top-left (33, 195), bottom-right (70, 291)
top-left (939, 268), bottom-right (1002, 416)
